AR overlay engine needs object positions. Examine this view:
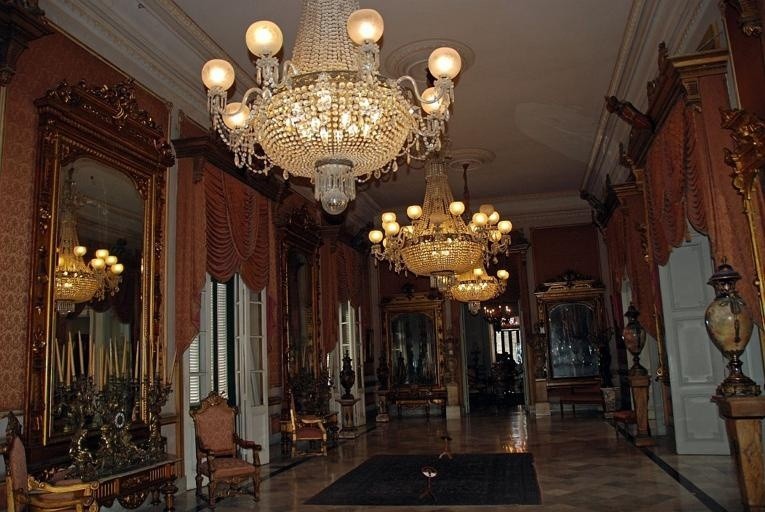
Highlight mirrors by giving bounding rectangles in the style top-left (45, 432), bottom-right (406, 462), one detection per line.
top-left (528, 280), bottom-right (606, 386)
top-left (379, 283), bottom-right (445, 390)
top-left (275, 202), bottom-right (324, 414)
top-left (23, 77), bottom-right (175, 478)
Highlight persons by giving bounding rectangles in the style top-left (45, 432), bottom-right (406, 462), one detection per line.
top-left (502, 352), bottom-right (517, 396)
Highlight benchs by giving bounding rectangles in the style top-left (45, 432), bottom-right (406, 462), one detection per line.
top-left (560, 392), bottom-right (606, 416)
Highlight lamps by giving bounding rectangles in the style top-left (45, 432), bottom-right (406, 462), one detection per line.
top-left (440, 164), bottom-right (509, 312)
top-left (201, 1), bottom-right (461, 216)
top-left (368, 66), bottom-right (495, 291)
top-left (52, 168), bottom-right (125, 317)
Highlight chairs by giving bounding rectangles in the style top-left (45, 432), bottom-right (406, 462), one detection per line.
top-left (288, 389), bottom-right (328, 458)
top-left (5, 410), bottom-right (100, 512)
top-left (612, 409), bottom-right (638, 442)
top-left (189, 392), bottom-right (262, 512)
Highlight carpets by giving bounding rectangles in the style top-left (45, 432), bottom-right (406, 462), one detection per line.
top-left (302, 453), bottom-right (542, 504)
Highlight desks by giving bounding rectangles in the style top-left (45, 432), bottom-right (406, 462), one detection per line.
top-left (35, 449), bottom-right (185, 512)
top-left (279, 411), bottom-right (339, 455)
top-left (396, 399), bottom-right (431, 416)
